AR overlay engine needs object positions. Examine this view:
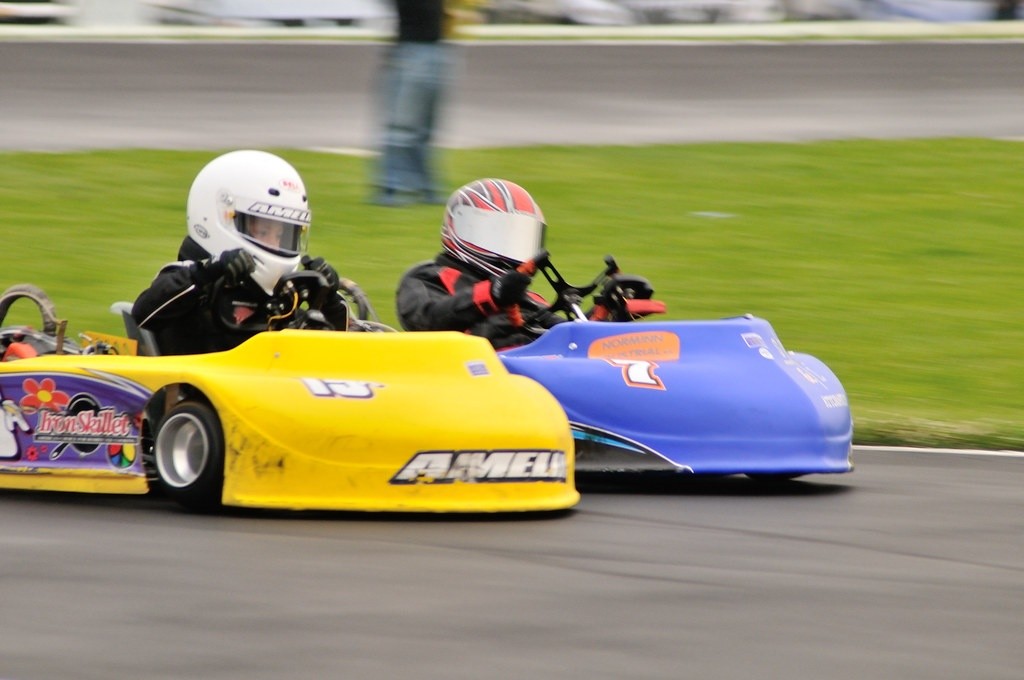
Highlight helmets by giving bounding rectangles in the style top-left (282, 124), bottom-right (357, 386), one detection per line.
top-left (187, 150), bottom-right (311, 296)
top-left (439, 178), bottom-right (547, 277)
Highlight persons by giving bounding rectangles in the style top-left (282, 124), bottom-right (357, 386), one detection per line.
top-left (132, 150), bottom-right (354, 354)
top-left (396, 178), bottom-right (635, 350)
top-left (372, 0), bottom-right (455, 207)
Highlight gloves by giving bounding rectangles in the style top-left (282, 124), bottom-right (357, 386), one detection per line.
top-left (189, 249), bottom-right (256, 299)
top-left (300, 255), bottom-right (340, 310)
top-left (600, 275), bottom-right (653, 322)
top-left (490, 271), bottom-right (532, 309)
